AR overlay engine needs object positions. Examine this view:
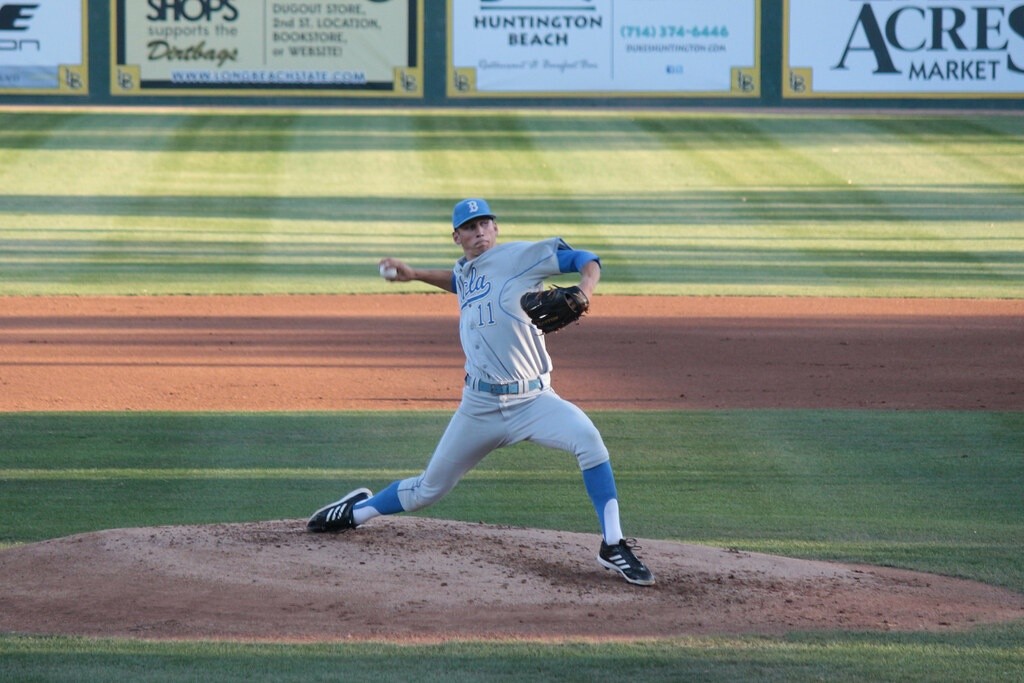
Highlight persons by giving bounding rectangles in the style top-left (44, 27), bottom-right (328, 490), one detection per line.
top-left (306, 200), bottom-right (656, 584)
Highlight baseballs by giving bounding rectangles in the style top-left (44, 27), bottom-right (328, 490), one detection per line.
top-left (379, 262), bottom-right (398, 282)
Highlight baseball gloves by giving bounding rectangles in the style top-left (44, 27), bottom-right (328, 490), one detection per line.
top-left (516, 281), bottom-right (588, 335)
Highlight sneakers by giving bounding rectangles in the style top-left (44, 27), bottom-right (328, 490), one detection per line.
top-left (597, 538), bottom-right (656, 585)
top-left (309, 488), bottom-right (373, 533)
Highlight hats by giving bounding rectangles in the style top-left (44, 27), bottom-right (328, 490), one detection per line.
top-left (452, 198), bottom-right (497, 229)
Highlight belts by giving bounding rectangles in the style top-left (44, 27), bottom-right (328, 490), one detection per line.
top-left (464, 374), bottom-right (541, 395)
